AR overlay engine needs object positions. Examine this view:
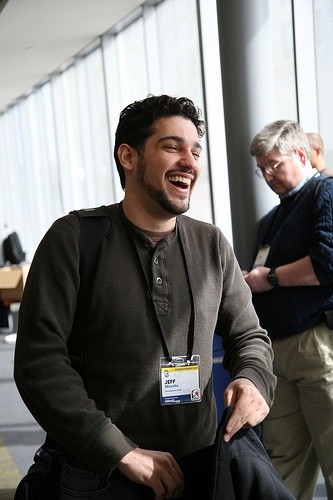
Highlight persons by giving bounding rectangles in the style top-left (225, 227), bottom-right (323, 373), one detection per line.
top-left (240, 118), bottom-right (333, 500)
top-left (305, 132), bottom-right (333, 179)
top-left (14, 96), bottom-right (277, 500)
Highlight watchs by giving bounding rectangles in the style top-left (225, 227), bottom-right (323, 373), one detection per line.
top-left (267, 265), bottom-right (280, 287)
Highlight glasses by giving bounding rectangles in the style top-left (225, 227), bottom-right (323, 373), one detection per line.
top-left (254, 150), bottom-right (296, 179)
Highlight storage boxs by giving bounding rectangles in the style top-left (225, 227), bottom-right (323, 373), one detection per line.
top-left (0, 266), bottom-right (28, 300)
